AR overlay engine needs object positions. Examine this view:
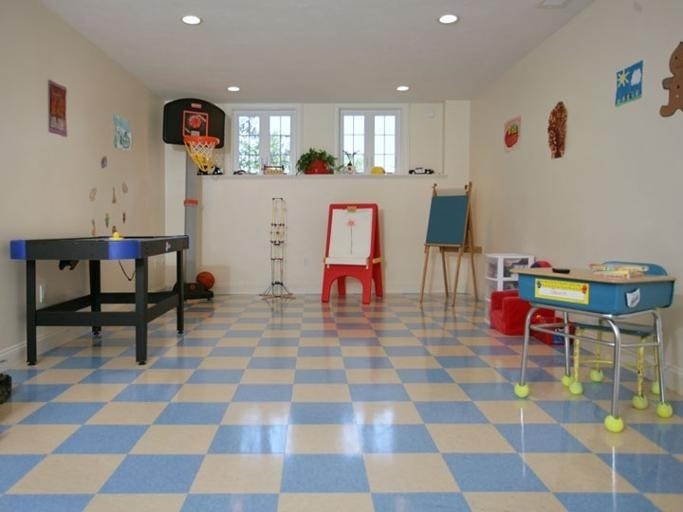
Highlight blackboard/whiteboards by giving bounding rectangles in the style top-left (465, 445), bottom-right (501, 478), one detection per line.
top-left (324, 207), bottom-right (374, 265)
top-left (426, 195), bottom-right (469, 248)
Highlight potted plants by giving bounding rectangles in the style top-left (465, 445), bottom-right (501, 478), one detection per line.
top-left (296, 147), bottom-right (344, 174)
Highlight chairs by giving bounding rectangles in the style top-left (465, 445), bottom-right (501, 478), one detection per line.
top-left (486, 258), bottom-right (555, 335)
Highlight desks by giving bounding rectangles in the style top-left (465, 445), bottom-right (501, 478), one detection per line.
top-left (8, 236), bottom-right (190, 367)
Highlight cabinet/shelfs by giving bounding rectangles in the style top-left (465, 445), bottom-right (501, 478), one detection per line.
top-left (482, 252), bottom-right (536, 326)
